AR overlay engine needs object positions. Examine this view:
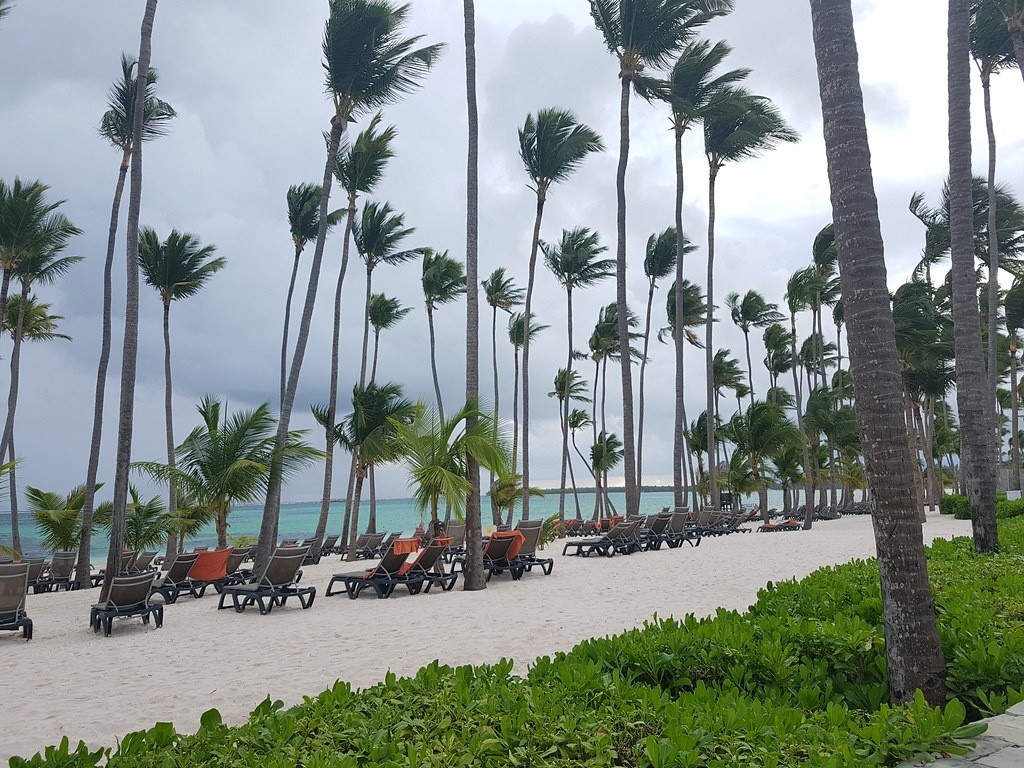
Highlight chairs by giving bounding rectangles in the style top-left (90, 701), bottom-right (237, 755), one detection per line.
top-left (557, 495), bottom-right (760, 558)
top-left (89, 537), bottom-right (319, 637)
top-left (757, 500), bottom-right (872, 533)
top-left (0, 552), bottom-right (76, 639)
top-left (319, 518), bottom-right (554, 600)
